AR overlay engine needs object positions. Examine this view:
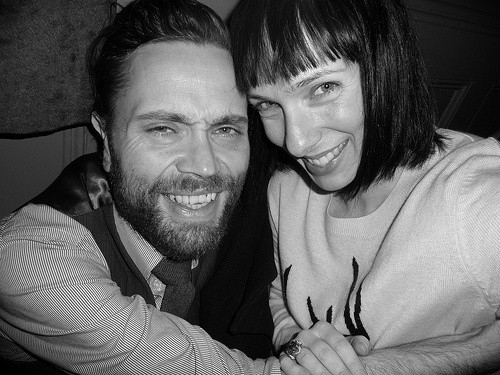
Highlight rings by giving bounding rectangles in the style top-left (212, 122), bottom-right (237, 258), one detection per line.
top-left (284, 339), bottom-right (307, 362)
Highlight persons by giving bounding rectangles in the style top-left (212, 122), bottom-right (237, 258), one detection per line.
top-left (229, 0), bottom-right (500, 374)
top-left (0, 0), bottom-right (499, 374)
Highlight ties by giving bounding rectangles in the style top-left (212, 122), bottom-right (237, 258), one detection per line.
top-left (152, 258), bottom-right (192, 319)
